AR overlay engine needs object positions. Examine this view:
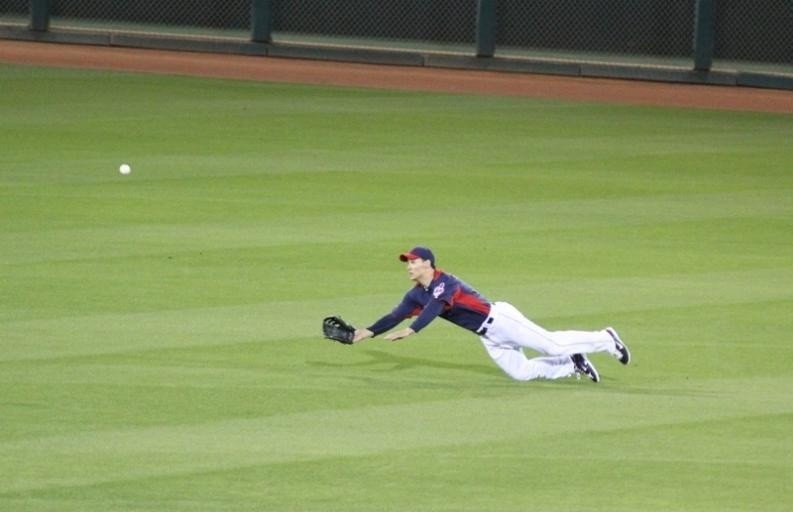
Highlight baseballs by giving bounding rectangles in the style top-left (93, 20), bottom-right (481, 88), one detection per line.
top-left (119, 164), bottom-right (131, 174)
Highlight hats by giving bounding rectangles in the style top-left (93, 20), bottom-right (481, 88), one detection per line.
top-left (399, 247), bottom-right (434, 262)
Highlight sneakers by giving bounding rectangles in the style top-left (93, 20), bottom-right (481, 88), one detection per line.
top-left (570, 353), bottom-right (599, 383)
top-left (604, 326), bottom-right (630, 365)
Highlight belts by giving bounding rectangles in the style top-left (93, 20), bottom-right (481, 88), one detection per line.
top-left (477, 318), bottom-right (494, 335)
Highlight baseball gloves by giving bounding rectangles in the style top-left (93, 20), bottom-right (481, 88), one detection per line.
top-left (323, 316), bottom-right (355, 346)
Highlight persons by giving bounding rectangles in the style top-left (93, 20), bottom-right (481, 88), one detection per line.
top-left (321, 245), bottom-right (629, 383)
